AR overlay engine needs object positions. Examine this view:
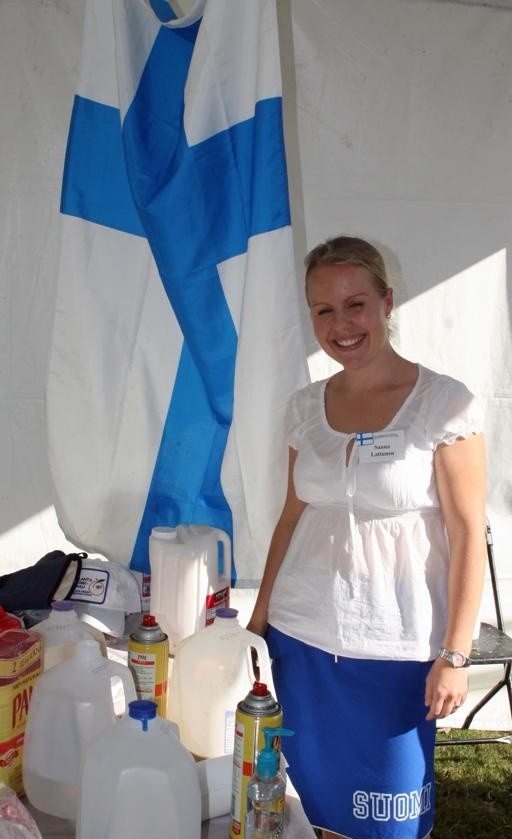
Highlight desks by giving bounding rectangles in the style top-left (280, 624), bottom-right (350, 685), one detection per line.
top-left (14, 776), bottom-right (318, 838)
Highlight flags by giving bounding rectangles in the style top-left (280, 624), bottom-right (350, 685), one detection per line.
top-left (35, 0), bottom-right (308, 592)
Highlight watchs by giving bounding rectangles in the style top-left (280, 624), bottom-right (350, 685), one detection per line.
top-left (438, 646), bottom-right (472, 670)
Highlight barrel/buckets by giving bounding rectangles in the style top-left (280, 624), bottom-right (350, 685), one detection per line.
top-left (77, 700), bottom-right (203, 839)
top-left (17, 639), bottom-right (138, 820)
top-left (145, 521), bottom-right (236, 654)
top-left (168, 603), bottom-right (280, 758)
top-left (26, 599), bottom-right (109, 672)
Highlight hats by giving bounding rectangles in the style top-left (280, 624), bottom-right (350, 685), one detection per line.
top-left (71, 559), bottom-right (141, 637)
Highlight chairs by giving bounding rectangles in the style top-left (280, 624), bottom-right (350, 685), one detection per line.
top-left (433, 515), bottom-right (512, 746)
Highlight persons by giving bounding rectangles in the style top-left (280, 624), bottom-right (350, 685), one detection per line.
top-left (242, 237), bottom-right (493, 838)
